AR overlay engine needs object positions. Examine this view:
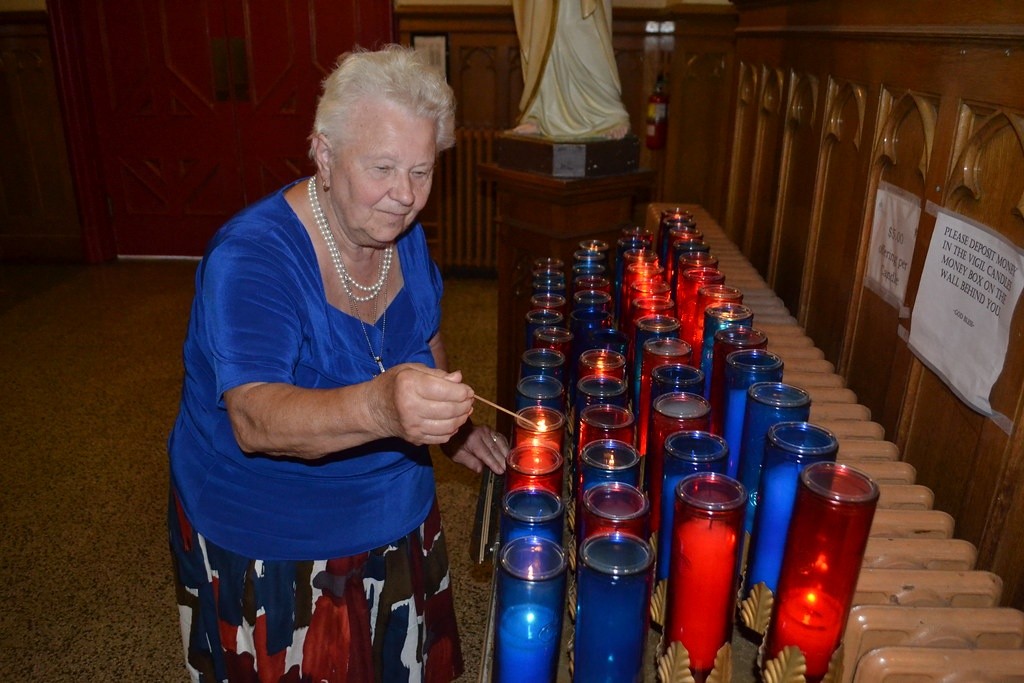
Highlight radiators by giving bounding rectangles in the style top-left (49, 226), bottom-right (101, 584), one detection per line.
top-left (646, 201), bottom-right (1024, 683)
top-left (435, 125), bottom-right (501, 269)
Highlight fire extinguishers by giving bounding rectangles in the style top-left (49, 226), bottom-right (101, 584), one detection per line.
top-left (644, 72), bottom-right (668, 148)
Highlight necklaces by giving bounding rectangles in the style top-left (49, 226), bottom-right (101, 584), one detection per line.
top-left (306, 175), bottom-right (392, 374)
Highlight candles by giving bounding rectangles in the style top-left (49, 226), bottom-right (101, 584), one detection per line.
top-left (490, 205), bottom-right (880, 683)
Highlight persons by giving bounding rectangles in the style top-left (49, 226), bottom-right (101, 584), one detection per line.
top-left (166, 41), bottom-right (512, 683)
top-left (502, 0), bottom-right (632, 140)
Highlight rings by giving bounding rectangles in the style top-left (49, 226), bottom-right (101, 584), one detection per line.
top-left (494, 433), bottom-right (499, 442)
